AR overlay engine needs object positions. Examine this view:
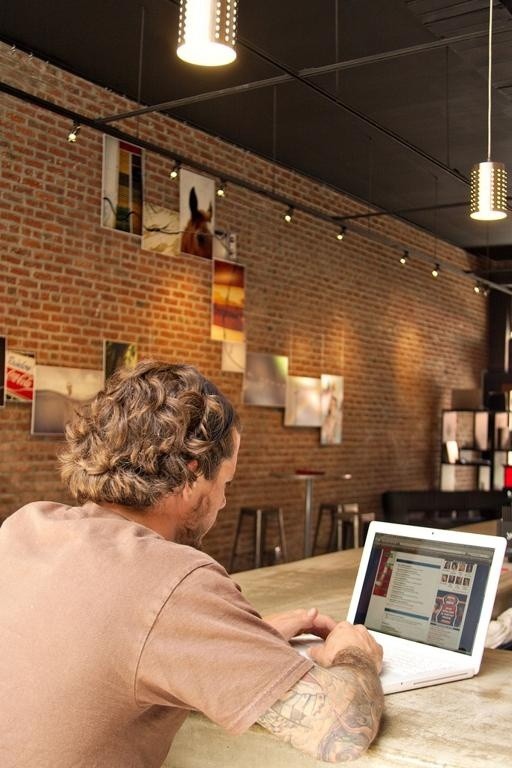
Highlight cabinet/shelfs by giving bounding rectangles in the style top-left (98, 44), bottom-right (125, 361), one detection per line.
top-left (439, 409), bottom-right (512, 493)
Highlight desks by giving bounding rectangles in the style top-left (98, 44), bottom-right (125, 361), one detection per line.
top-left (269, 470), bottom-right (352, 558)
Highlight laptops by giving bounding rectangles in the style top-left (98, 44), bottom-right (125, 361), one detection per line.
top-left (287, 519), bottom-right (508, 695)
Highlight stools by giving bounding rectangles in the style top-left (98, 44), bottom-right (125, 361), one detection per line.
top-left (231, 505), bottom-right (288, 572)
top-left (313, 501), bottom-right (359, 555)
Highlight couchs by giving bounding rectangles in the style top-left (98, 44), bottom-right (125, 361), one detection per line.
top-left (381, 489), bottom-right (512, 529)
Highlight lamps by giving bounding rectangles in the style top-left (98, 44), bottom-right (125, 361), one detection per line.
top-left (432, 268), bottom-right (439, 277)
top-left (474, 283), bottom-right (491, 297)
top-left (176, 0), bottom-right (240, 66)
top-left (170, 165), bottom-right (180, 178)
top-left (400, 256), bottom-right (407, 264)
top-left (336, 230), bottom-right (345, 240)
top-left (217, 183), bottom-right (225, 196)
top-left (469, 0), bottom-right (509, 222)
top-left (66, 122), bottom-right (80, 143)
top-left (284, 210), bottom-right (293, 223)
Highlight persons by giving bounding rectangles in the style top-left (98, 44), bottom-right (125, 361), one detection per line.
top-left (0, 357), bottom-right (384, 768)
